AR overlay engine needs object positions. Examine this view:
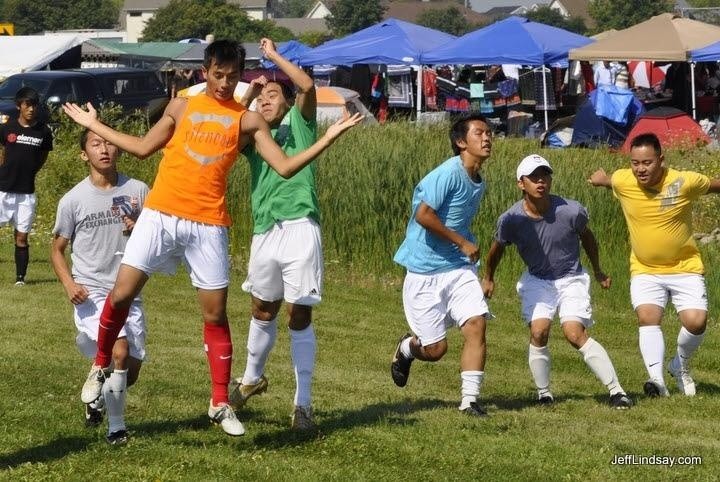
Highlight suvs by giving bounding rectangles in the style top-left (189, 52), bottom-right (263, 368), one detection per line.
top-left (0, 67), bottom-right (168, 130)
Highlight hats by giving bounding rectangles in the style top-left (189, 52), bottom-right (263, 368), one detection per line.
top-left (516, 153), bottom-right (554, 181)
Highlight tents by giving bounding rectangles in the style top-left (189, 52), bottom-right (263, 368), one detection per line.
top-left (297, 19), bottom-right (460, 129)
top-left (566, 13), bottom-right (720, 117)
top-left (574, 85), bottom-right (648, 148)
top-left (312, 85), bottom-right (379, 130)
top-left (686, 42), bottom-right (720, 125)
top-left (621, 105), bottom-right (712, 158)
top-left (254, 39), bottom-right (311, 83)
top-left (413, 17), bottom-right (595, 135)
top-left (0, 35), bottom-right (94, 83)
top-left (174, 80), bottom-right (257, 115)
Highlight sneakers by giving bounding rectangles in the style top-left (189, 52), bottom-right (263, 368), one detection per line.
top-left (666, 359), bottom-right (697, 396)
top-left (643, 379), bottom-right (670, 398)
top-left (390, 332), bottom-right (415, 388)
top-left (106, 429), bottom-right (129, 446)
top-left (289, 403), bottom-right (313, 437)
top-left (14, 276), bottom-right (25, 286)
top-left (458, 401), bottom-right (491, 419)
top-left (227, 374), bottom-right (269, 415)
top-left (84, 403), bottom-right (105, 428)
top-left (207, 397), bottom-right (246, 437)
top-left (80, 360), bottom-right (114, 405)
top-left (608, 392), bottom-right (634, 409)
top-left (537, 392), bottom-right (555, 404)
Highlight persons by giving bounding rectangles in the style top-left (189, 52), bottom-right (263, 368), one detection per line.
top-left (612, 61), bottom-right (629, 87)
top-left (0, 88), bottom-right (52, 285)
top-left (228, 37), bottom-right (323, 433)
top-left (588, 134), bottom-right (720, 399)
top-left (391, 110), bottom-right (495, 422)
top-left (594, 61), bottom-right (616, 86)
top-left (62, 39), bottom-right (367, 436)
top-left (482, 153), bottom-right (635, 412)
top-left (51, 121), bottom-right (151, 449)
